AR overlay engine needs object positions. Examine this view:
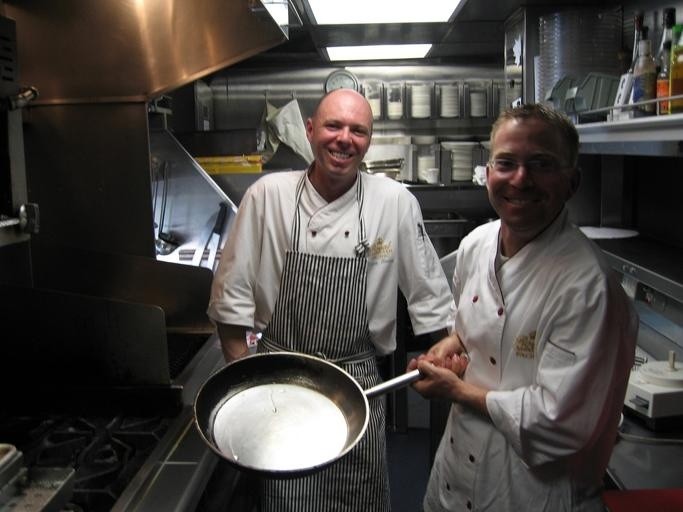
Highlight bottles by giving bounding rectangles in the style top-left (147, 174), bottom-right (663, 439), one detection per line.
top-left (631, 7), bottom-right (682, 117)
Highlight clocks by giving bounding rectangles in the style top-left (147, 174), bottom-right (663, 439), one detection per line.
top-left (324, 70), bottom-right (360, 94)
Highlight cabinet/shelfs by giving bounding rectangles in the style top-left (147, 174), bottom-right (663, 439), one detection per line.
top-left (355, 111), bottom-right (501, 212)
top-left (522, 0), bottom-right (683, 160)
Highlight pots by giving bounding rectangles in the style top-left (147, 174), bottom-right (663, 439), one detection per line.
top-left (194, 351), bottom-right (427, 479)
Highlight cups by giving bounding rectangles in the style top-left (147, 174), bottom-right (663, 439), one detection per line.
top-left (422, 168), bottom-right (439, 184)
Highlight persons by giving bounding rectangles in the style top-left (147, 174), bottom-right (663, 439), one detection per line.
top-left (405, 103), bottom-right (639, 511)
top-left (207, 86), bottom-right (458, 511)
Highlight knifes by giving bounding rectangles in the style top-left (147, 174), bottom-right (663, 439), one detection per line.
top-left (205, 202), bottom-right (227, 272)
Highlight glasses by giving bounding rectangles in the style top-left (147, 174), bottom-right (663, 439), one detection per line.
top-left (484, 159), bottom-right (578, 174)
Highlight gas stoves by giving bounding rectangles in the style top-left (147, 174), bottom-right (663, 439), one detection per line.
top-left (0, 381), bottom-right (218, 512)
